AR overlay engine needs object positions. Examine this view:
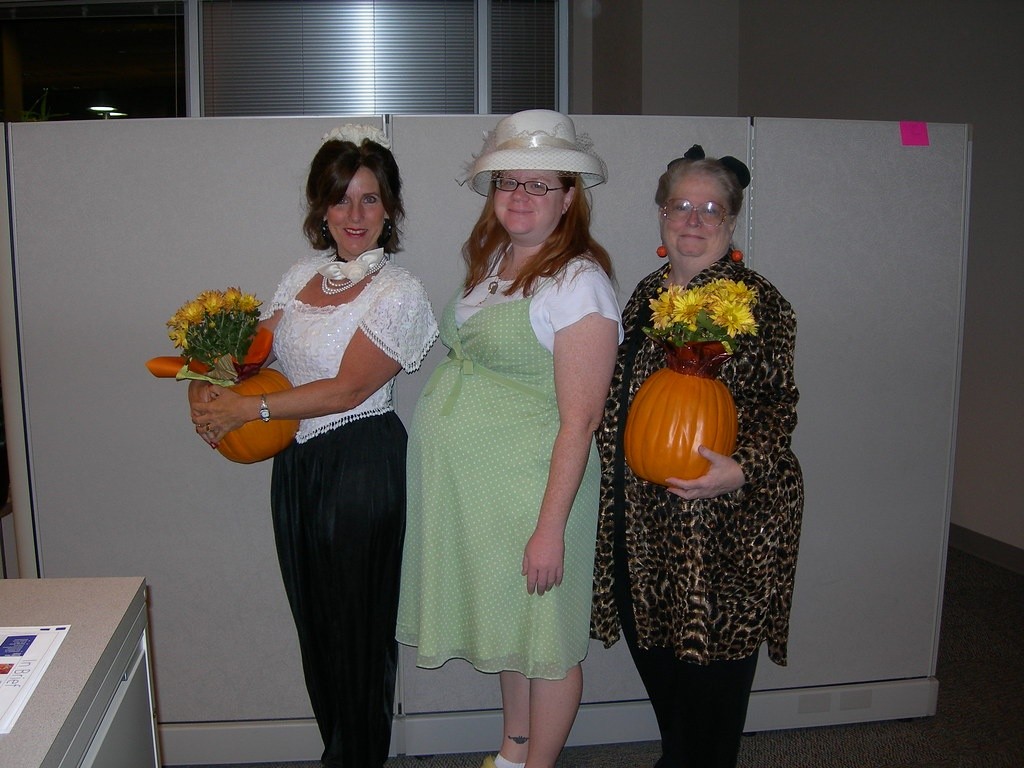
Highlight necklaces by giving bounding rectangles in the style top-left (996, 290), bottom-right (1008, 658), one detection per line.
top-left (475, 250), bottom-right (521, 307)
top-left (322, 247), bottom-right (389, 295)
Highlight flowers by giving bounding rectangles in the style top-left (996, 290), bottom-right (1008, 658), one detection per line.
top-left (167, 284), bottom-right (263, 382)
top-left (645, 278), bottom-right (765, 372)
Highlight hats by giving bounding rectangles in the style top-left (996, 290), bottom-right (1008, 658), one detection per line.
top-left (455, 110), bottom-right (608, 192)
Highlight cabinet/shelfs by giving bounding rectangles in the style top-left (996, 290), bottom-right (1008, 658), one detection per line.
top-left (0, 577), bottom-right (165, 768)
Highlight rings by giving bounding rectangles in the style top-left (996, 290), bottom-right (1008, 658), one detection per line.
top-left (205, 424), bottom-right (210, 433)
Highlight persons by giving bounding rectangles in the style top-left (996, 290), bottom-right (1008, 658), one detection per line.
top-left (589, 145), bottom-right (803, 768)
top-left (194, 123), bottom-right (439, 768)
top-left (393, 110), bottom-right (624, 768)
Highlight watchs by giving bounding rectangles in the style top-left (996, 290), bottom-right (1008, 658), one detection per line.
top-left (259, 393), bottom-right (271, 423)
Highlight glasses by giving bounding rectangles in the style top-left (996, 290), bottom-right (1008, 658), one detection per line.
top-left (658, 196), bottom-right (733, 228)
top-left (487, 178), bottom-right (567, 196)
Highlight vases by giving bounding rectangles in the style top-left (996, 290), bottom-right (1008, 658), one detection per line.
top-left (624, 367), bottom-right (737, 487)
top-left (210, 368), bottom-right (299, 464)
